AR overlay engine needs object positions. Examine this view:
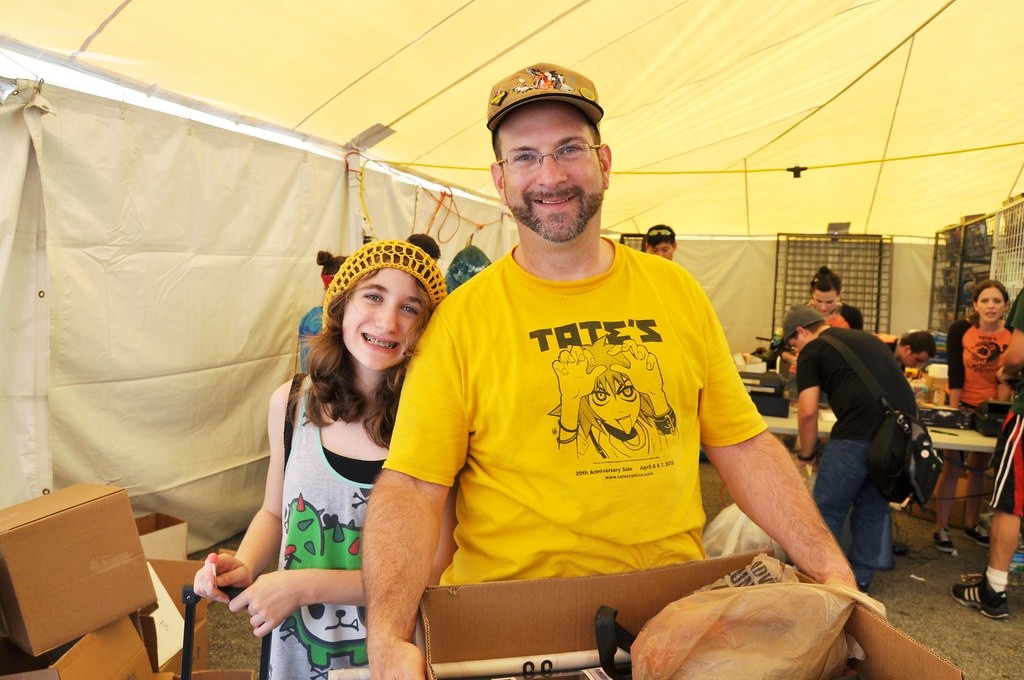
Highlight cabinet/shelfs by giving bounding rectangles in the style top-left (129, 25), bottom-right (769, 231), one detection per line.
top-left (926, 215), bottom-right (995, 338)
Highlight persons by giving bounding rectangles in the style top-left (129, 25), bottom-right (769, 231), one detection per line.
top-left (406, 234), bottom-right (441, 263)
top-left (645, 225), bottom-right (678, 261)
top-left (783, 302), bottom-right (918, 594)
top-left (780, 268), bottom-right (864, 405)
top-left (361, 64), bottom-right (856, 680)
top-left (193, 241), bottom-right (449, 680)
top-left (933, 280), bottom-right (1011, 550)
top-left (445, 246), bottom-right (492, 294)
top-left (952, 291), bottom-right (1024, 618)
top-left (886, 331), bottom-right (936, 375)
top-left (299, 250), bottom-right (348, 375)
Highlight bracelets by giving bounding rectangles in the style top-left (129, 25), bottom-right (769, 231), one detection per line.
top-left (797, 451), bottom-right (816, 461)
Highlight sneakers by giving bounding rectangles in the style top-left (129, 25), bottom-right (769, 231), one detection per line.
top-left (933, 528), bottom-right (954, 552)
top-left (964, 523), bottom-right (990, 546)
top-left (952, 567), bottom-right (1009, 618)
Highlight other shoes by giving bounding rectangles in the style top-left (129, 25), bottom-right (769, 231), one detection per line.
top-left (893, 541), bottom-right (907, 554)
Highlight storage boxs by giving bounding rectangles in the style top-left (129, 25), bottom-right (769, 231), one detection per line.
top-left (0, 481), bottom-right (254, 680)
top-left (417, 546), bottom-right (967, 680)
top-left (908, 468), bottom-right (984, 531)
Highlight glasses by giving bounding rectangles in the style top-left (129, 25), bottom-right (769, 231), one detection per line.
top-left (649, 229), bottom-right (671, 236)
top-left (497, 142), bottom-right (600, 172)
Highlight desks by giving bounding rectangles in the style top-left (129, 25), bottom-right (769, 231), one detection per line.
top-left (755, 408), bottom-right (998, 454)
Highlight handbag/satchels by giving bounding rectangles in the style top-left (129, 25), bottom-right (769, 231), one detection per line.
top-left (870, 410), bottom-right (942, 508)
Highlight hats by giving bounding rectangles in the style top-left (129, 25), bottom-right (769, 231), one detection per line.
top-left (487, 63), bottom-right (604, 132)
top-left (775, 306), bottom-right (822, 348)
